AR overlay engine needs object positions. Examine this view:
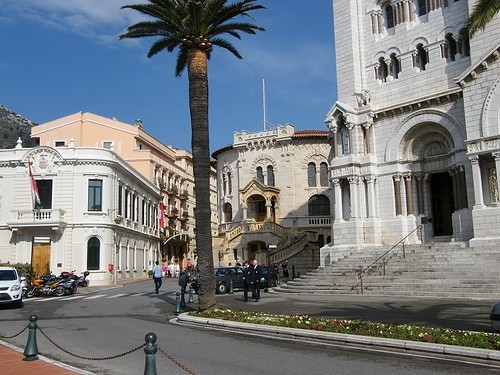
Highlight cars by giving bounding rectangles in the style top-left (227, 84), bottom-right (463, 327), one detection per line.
top-left (191, 266), bottom-right (245, 294)
top-left (259, 265), bottom-right (280, 288)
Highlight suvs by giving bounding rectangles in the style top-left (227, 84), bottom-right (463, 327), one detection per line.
top-left (0, 266), bottom-right (26, 307)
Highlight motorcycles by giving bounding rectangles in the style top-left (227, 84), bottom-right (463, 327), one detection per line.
top-left (21, 269), bottom-right (90, 298)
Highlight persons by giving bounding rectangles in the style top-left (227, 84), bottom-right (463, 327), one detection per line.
top-left (153, 261), bottom-right (162, 295)
top-left (228, 261), bottom-right (249, 273)
top-left (274, 261), bottom-right (289, 284)
top-left (243, 259), bottom-right (261, 301)
top-left (162, 263), bottom-right (173, 277)
top-left (178, 263), bottom-right (192, 306)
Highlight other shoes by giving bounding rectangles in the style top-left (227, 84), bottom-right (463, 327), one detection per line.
top-left (155, 288), bottom-right (160, 294)
top-left (244, 298), bottom-right (248, 302)
top-left (255, 298), bottom-right (260, 302)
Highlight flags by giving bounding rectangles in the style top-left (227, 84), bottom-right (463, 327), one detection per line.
top-left (159, 203), bottom-right (164, 232)
top-left (29, 168), bottom-right (39, 205)
